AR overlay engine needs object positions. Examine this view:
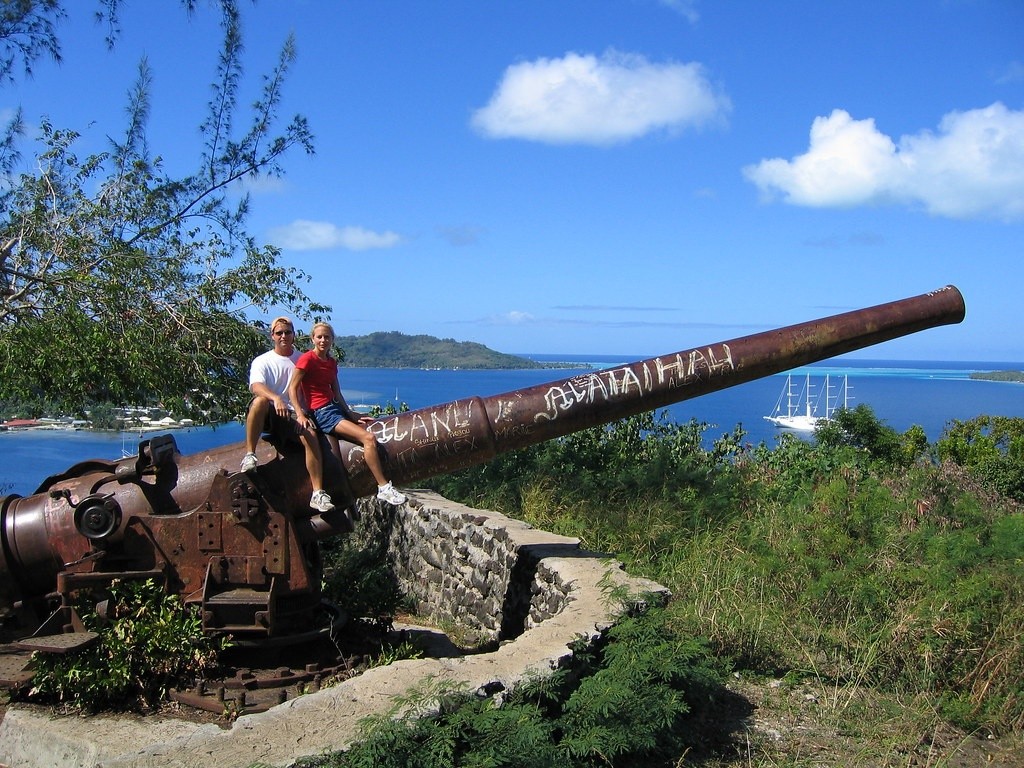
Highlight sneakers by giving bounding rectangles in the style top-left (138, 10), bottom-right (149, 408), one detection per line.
top-left (310, 490), bottom-right (336, 512)
top-left (376, 480), bottom-right (407, 505)
top-left (240, 454), bottom-right (258, 472)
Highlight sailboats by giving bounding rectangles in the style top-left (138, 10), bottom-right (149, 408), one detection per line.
top-left (762, 373), bottom-right (856, 435)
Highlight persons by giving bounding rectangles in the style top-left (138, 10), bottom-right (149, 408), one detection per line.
top-left (239, 315), bottom-right (338, 513)
top-left (288, 322), bottom-right (410, 506)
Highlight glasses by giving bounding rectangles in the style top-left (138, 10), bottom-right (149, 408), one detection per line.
top-left (272, 330), bottom-right (293, 336)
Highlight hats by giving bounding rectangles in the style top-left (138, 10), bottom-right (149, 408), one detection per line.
top-left (271, 317), bottom-right (294, 334)
